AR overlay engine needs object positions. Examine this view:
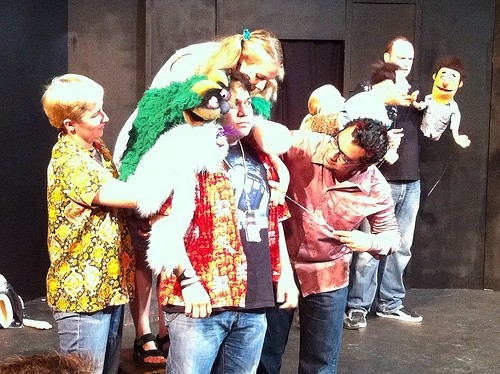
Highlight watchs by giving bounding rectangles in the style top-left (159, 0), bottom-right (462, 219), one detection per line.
top-left (178, 267), bottom-right (196, 282)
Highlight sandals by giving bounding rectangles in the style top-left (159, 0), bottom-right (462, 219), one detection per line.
top-left (132, 333), bottom-right (170, 369)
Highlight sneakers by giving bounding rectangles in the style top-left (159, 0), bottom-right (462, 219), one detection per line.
top-left (342, 306), bottom-right (369, 331)
top-left (375, 304), bottom-right (423, 322)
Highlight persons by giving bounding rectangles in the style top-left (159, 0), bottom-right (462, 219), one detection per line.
top-left (343, 35), bottom-right (422, 329)
top-left (345, 61), bottom-right (411, 128)
top-left (40, 30), bottom-right (404, 374)
top-left (411, 57), bottom-right (471, 163)
top-left (0, 348), bottom-right (97, 374)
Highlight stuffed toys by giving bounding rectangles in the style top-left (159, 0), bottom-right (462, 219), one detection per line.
top-left (118, 75), bottom-right (230, 184)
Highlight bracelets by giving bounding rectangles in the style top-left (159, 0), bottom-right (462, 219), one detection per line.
top-left (181, 276), bottom-right (199, 289)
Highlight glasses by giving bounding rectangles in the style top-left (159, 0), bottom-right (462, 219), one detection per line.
top-left (329, 126), bottom-right (363, 167)
